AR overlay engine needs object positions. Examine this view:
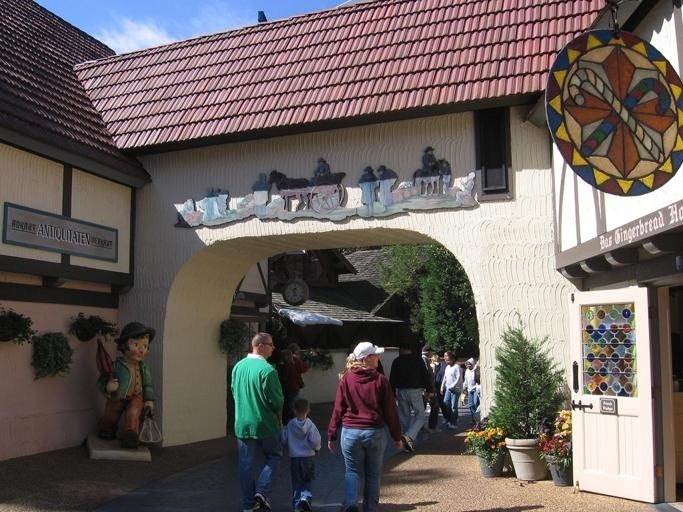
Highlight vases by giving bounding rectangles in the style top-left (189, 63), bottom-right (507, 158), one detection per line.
top-left (543, 455), bottom-right (573, 487)
top-left (476, 454), bottom-right (504, 478)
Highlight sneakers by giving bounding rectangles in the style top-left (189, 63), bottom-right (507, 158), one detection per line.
top-left (401, 433), bottom-right (415, 452)
top-left (293, 494), bottom-right (313, 512)
top-left (243, 492), bottom-right (270, 512)
top-left (427, 417), bottom-right (459, 433)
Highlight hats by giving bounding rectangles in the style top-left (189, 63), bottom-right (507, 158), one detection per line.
top-left (422, 345), bottom-right (435, 352)
top-left (354, 342), bottom-right (385, 361)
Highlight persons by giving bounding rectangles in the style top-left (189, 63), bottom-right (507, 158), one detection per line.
top-left (104, 316), bottom-right (156, 452)
top-left (326, 340), bottom-right (403, 511)
top-left (275, 349), bottom-right (302, 425)
top-left (389, 343), bottom-right (486, 450)
top-left (231, 328), bottom-right (282, 512)
top-left (284, 395), bottom-right (322, 512)
top-left (280, 343), bottom-right (313, 376)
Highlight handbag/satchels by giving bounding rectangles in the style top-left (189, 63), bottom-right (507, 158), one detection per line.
top-left (453, 386), bottom-right (461, 395)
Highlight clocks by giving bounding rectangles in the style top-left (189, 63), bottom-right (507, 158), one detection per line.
top-left (282, 278), bottom-right (309, 306)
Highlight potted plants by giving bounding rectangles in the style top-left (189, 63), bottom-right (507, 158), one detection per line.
top-left (487, 321), bottom-right (565, 481)
top-left (69, 312), bottom-right (120, 341)
top-left (0, 303), bottom-right (38, 346)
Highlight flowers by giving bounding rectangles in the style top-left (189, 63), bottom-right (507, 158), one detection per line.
top-left (536, 410), bottom-right (572, 467)
top-left (461, 425), bottom-right (510, 469)
